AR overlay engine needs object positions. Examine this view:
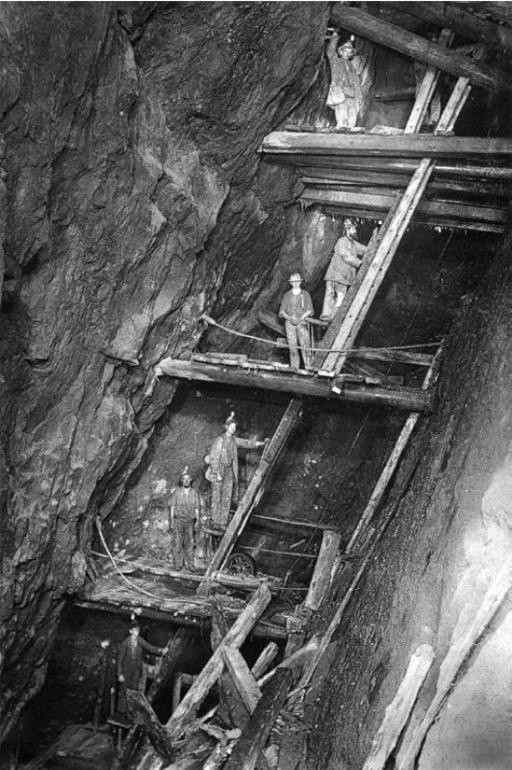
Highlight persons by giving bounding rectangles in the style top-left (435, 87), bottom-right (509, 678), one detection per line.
top-left (277, 273), bottom-right (317, 373)
top-left (323, 29), bottom-right (365, 131)
top-left (117, 621), bottom-right (168, 726)
top-left (319, 217), bottom-right (371, 322)
top-left (202, 417), bottom-right (267, 532)
top-left (168, 472), bottom-right (203, 571)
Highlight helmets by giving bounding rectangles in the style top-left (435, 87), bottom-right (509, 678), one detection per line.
top-left (288, 274), bottom-right (304, 282)
top-left (338, 42), bottom-right (357, 56)
